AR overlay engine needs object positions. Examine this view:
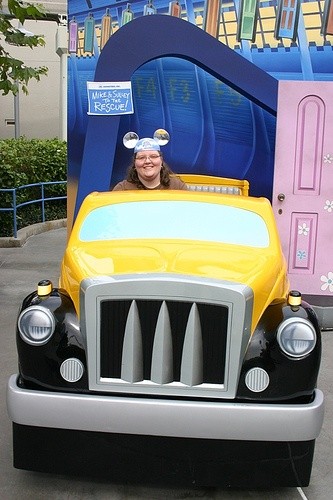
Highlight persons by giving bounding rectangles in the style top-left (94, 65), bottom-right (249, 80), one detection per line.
top-left (112, 137), bottom-right (187, 191)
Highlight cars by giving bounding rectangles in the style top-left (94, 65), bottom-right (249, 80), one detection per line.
top-left (6, 171), bottom-right (325, 486)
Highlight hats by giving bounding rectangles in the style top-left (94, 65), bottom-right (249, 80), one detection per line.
top-left (122, 128), bottom-right (170, 153)
